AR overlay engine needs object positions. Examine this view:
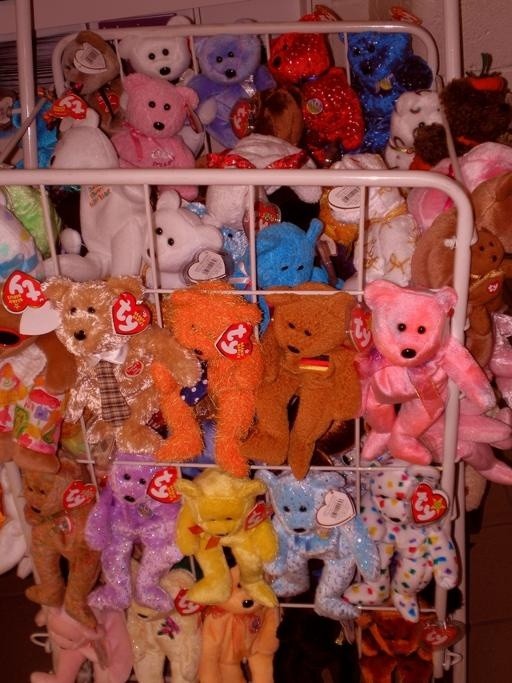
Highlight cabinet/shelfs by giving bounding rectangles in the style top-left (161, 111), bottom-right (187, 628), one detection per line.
top-left (1, 0), bottom-right (474, 683)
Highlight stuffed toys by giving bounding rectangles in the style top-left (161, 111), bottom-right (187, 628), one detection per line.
top-left (0, 7), bottom-right (512, 682)
top-left (2, 8), bottom-right (512, 682)
